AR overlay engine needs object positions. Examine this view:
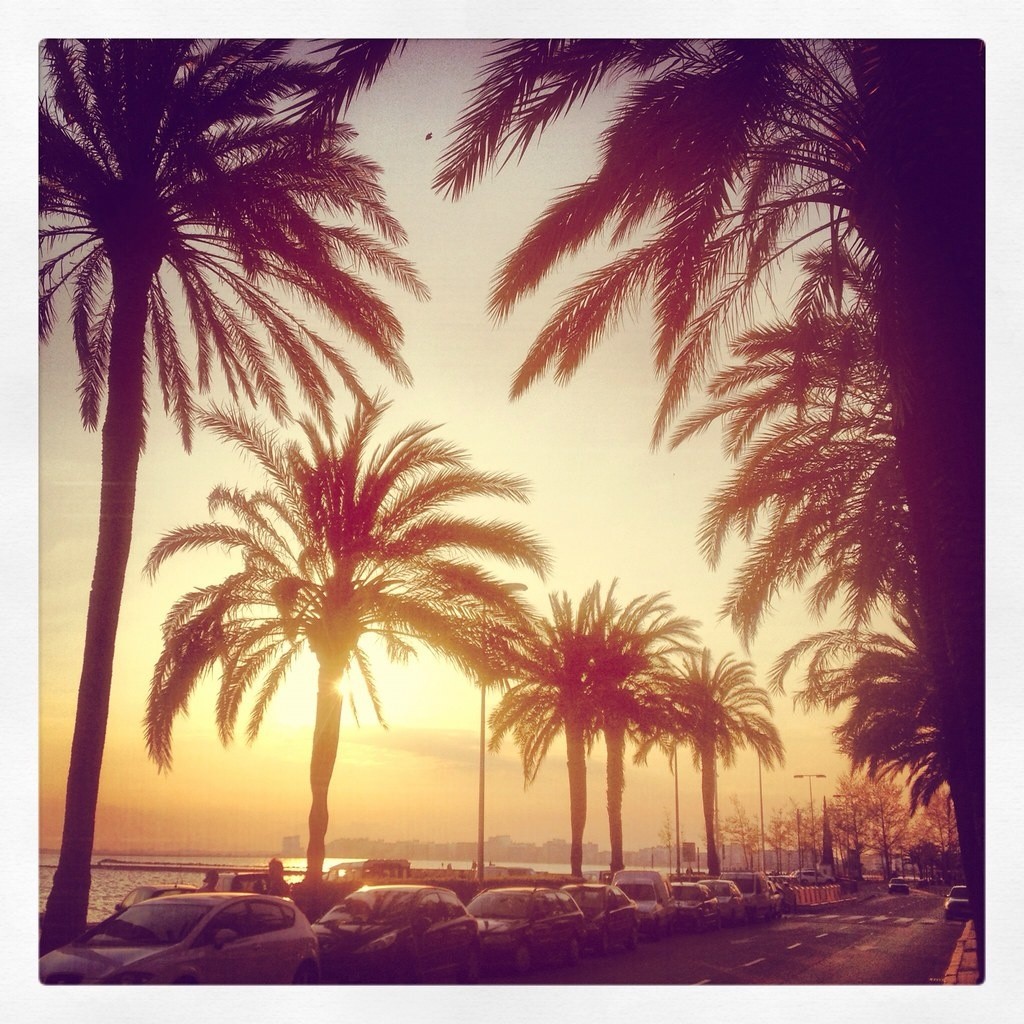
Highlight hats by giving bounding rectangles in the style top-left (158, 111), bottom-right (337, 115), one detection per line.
top-left (203, 869), bottom-right (219, 882)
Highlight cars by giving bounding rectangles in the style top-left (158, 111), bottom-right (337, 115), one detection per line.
top-left (196, 872), bottom-right (279, 896)
top-left (558, 882), bottom-right (641, 957)
top-left (668, 881), bottom-right (722, 934)
top-left (888, 877), bottom-right (909, 895)
top-left (461, 885), bottom-right (586, 978)
top-left (111, 884), bottom-right (197, 921)
top-left (694, 879), bottom-right (751, 927)
top-left (789, 867), bottom-right (836, 887)
top-left (309, 884), bottom-right (478, 986)
top-left (608, 869), bottom-right (676, 935)
top-left (942, 885), bottom-right (971, 921)
top-left (39, 891), bottom-right (323, 985)
top-left (716, 871), bottom-right (784, 921)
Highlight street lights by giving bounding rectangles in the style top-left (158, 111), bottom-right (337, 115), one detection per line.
top-left (793, 774), bottom-right (827, 885)
top-left (443, 581), bottom-right (529, 884)
top-left (833, 795), bottom-right (859, 879)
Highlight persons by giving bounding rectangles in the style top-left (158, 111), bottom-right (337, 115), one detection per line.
top-left (196, 869), bottom-right (220, 893)
top-left (440, 859), bottom-right (491, 871)
top-left (267, 857), bottom-right (293, 897)
